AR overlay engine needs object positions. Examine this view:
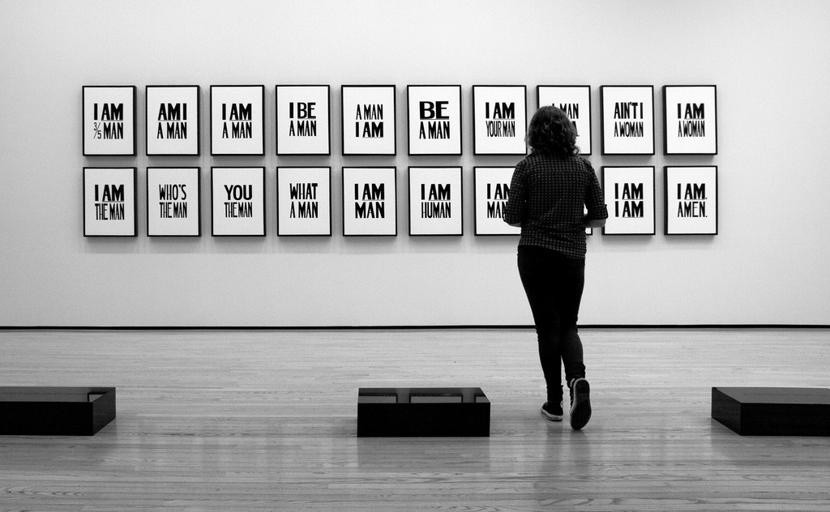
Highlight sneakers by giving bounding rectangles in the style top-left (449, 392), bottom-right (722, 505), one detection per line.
top-left (541, 401), bottom-right (563, 422)
top-left (568, 377), bottom-right (592, 430)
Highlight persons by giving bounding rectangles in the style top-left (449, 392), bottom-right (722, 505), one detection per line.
top-left (501, 105), bottom-right (610, 431)
